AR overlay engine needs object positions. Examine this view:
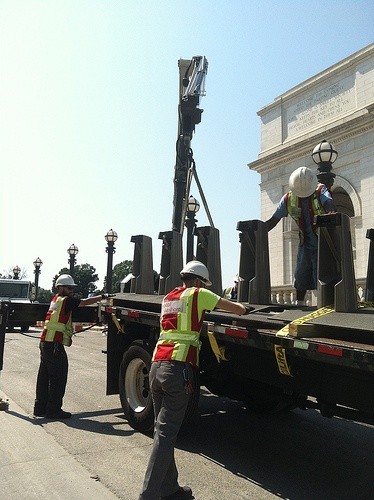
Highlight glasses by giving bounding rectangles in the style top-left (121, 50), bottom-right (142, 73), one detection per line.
top-left (64, 286), bottom-right (74, 289)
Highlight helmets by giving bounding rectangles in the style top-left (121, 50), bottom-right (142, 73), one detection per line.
top-left (55, 274), bottom-right (77, 287)
top-left (180, 260), bottom-right (212, 286)
top-left (289, 167), bottom-right (318, 198)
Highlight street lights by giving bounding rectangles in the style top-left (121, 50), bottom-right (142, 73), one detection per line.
top-left (104, 229), bottom-right (118, 294)
top-left (313, 140), bottom-right (338, 193)
top-left (13, 265), bottom-right (21, 279)
top-left (185, 195), bottom-right (199, 262)
top-left (67, 244), bottom-right (79, 275)
top-left (33, 257), bottom-right (43, 301)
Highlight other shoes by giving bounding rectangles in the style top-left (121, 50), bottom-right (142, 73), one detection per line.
top-left (48, 411), bottom-right (71, 418)
top-left (34, 409), bottom-right (49, 416)
top-left (163, 486), bottom-right (192, 500)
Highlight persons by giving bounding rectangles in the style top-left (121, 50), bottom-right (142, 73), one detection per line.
top-left (33, 274), bottom-right (116, 419)
top-left (264, 166), bottom-right (336, 306)
top-left (139, 260), bottom-right (255, 500)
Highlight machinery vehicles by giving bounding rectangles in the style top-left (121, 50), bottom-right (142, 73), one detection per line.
top-left (0, 278), bottom-right (50, 332)
top-left (102, 213), bottom-right (373, 428)
